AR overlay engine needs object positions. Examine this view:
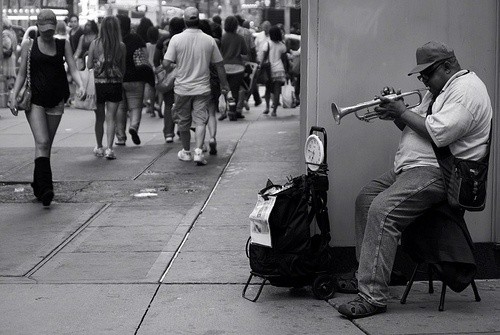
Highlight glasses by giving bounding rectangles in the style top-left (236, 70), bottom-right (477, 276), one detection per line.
top-left (417, 61), bottom-right (445, 86)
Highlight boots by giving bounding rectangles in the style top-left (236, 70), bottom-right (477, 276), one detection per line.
top-left (31, 157), bottom-right (54, 206)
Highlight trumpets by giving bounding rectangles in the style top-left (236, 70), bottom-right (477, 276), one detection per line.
top-left (330, 84), bottom-right (431, 126)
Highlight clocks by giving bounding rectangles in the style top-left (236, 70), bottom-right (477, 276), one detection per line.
top-left (304, 135), bottom-right (324, 172)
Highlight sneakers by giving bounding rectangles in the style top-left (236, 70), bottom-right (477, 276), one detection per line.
top-left (177, 149), bottom-right (192, 161)
top-left (193, 149), bottom-right (208, 165)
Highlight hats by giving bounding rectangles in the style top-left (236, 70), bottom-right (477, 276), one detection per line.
top-left (184, 6), bottom-right (199, 22)
top-left (407, 41), bottom-right (455, 76)
top-left (37, 9), bottom-right (57, 32)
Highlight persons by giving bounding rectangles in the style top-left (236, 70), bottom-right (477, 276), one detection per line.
top-left (9, 10), bottom-right (87, 206)
top-left (0, 9), bottom-right (40, 101)
top-left (332, 41), bottom-right (494, 318)
top-left (55, 5), bottom-right (301, 165)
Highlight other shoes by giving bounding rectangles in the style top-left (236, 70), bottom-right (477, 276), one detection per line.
top-left (209, 138), bottom-right (217, 155)
top-left (165, 136), bottom-right (173, 143)
top-left (202, 143), bottom-right (207, 151)
top-left (146, 99), bottom-right (151, 113)
top-left (115, 136), bottom-right (126, 145)
top-left (128, 128), bottom-right (140, 144)
top-left (105, 148), bottom-right (116, 159)
top-left (93, 146), bottom-right (103, 157)
top-left (218, 100), bottom-right (277, 121)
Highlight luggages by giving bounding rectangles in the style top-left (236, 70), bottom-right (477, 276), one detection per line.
top-left (242, 126), bottom-right (333, 301)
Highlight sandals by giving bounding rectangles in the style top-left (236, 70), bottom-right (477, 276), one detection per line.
top-left (339, 296), bottom-right (386, 319)
top-left (334, 278), bottom-right (359, 293)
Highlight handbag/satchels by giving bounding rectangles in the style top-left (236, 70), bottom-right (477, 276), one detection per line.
top-left (7, 85), bottom-right (31, 111)
top-left (256, 41), bottom-right (271, 85)
top-left (280, 78), bottom-right (297, 108)
top-left (438, 155), bottom-right (486, 212)
top-left (74, 64), bottom-right (98, 110)
